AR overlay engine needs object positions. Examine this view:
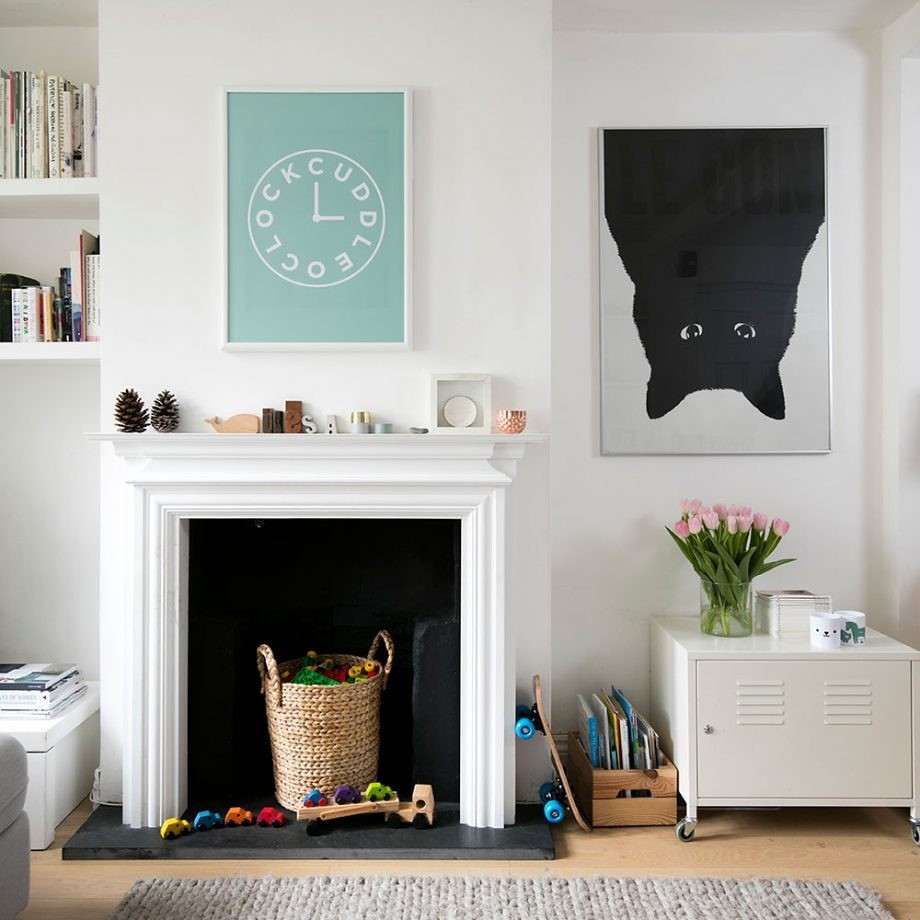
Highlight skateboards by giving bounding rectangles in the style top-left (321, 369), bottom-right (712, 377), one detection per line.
top-left (515, 674), bottom-right (594, 834)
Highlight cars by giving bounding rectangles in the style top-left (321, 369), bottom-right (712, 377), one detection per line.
top-left (257, 806), bottom-right (286, 828)
top-left (365, 782), bottom-right (393, 801)
top-left (223, 807), bottom-right (253, 828)
top-left (304, 789), bottom-right (329, 807)
top-left (336, 786), bottom-right (362, 804)
top-left (193, 810), bottom-right (224, 832)
top-left (159, 818), bottom-right (192, 840)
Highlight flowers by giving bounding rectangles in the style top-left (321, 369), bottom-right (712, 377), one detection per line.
top-left (664, 499), bottom-right (798, 637)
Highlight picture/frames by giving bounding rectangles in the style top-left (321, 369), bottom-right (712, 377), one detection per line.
top-left (428, 372), bottom-right (492, 436)
top-left (217, 87), bottom-right (415, 352)
top-left (598, 124), bottom-right (831, 455)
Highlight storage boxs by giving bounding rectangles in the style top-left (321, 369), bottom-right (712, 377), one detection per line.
top-left (565, 730), bottom-right (679, 828)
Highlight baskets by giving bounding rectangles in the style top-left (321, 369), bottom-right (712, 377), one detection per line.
top-left (256, 629), bottom-right (395, 811)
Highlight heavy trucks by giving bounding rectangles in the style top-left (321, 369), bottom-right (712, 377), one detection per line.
top-left (296, 783), bottom-right (437, 836)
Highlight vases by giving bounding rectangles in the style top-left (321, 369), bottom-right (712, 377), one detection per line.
top-left (699, 579), bottom-right (754, 638)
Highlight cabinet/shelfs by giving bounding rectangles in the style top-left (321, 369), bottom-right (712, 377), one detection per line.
top-left (647, 613), bottom-right (920, 847)
top-left (0, 0), bottom-right (99, 362)
top-left (1, 679), bottom-right (99, 850)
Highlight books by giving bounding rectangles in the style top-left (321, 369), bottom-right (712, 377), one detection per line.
top-left (0, 62), bottom-right (100, 181)
top-left (0, 223), bottom-right (99, 347)
top-left (754, 587), bottom-right (836, 640)
top-left (574, 682), bottom-right (665, 799)
top-left (0, 660), bottom-right (94, 721)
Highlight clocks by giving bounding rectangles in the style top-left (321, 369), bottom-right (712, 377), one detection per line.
top-left (246, 150), bottom-right (387, 287)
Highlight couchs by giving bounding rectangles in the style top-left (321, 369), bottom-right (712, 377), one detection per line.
top-left (0, 730), bottom-right (30, 920)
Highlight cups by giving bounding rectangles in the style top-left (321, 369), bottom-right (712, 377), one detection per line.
top-left (835, 610), bottom-right (866, 646)
top-left (810, 611), bottom-right (843, 650)
top-left (497, 407), bottom-right (527, 434)
